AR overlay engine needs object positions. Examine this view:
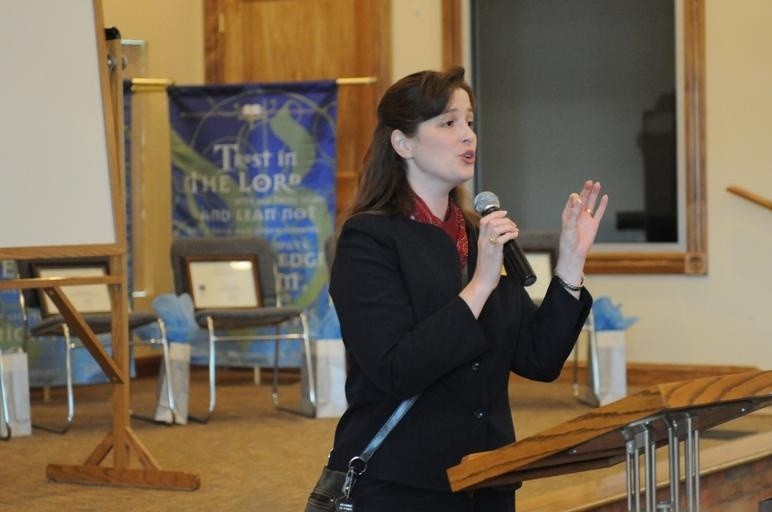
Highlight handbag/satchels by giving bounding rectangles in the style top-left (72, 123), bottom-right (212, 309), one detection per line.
top-left (304, 467), bottom-right (359, 512)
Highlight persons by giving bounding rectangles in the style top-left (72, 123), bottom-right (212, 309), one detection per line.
top-left (306, 67), bottom-right (612, 512)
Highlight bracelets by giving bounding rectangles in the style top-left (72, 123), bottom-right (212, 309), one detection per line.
top-left (552, 269), bottom-right (587, 291)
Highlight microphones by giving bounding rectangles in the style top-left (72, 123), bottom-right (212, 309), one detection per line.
top-left (473, 191), bottom-right (537, 287)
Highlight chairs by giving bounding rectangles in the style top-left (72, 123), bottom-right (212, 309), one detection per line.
top-left (15, 251), bottom-right (173, 433)
top-left (161, 239), bottom-right (318, 423)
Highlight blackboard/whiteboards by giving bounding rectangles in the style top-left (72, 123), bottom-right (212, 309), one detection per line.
top-left (0, 0), bottom-right (129, 262)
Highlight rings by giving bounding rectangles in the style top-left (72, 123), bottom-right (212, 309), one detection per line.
top-left (585, 207), bottom-right (594, 216)
top-left (489, 236), bottom-right (496, 245)
